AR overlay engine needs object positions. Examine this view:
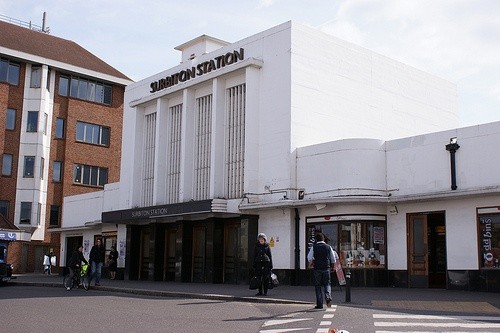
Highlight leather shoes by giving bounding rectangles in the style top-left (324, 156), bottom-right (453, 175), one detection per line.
top-left (326, 299), bottom-right (332, 307)
top-left (315, 303), bottom-right (323, 309)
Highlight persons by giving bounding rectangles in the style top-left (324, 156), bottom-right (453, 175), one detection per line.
top-left (42, 252), bottom-right (52, 275)
top-left (307, 233), bottom-right (336, 309)
top-left (108, 246), bottom-right (118, 280)
top-left (253, 233), bottom-right (272, 296)
top-left (88, 238), bottom-right (106, 286)
top-left (66, 244), bottom-right (88, 290)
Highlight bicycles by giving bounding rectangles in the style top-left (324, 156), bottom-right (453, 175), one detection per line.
top-left (63, 266), bottom-right (90, 291)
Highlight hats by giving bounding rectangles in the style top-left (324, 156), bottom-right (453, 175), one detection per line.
top-left (257, 233), bottom-right (267, 241)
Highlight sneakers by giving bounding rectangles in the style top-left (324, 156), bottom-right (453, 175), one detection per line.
top-left (67, 287), bottom-right (70, 290)
top-left (95, 283), bottom-right (100, 286)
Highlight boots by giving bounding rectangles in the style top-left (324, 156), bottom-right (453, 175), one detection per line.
top-left (256, 287), bottom-right (263, 296)
top-left (262, 287), bottom-right (267, 296)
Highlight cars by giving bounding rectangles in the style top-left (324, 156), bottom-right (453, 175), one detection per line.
top-left (0, 263), bottom-right (13, 282)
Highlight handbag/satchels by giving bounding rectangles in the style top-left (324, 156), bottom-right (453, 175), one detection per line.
top-left (248, 272), bottom-right (256, 290)
top-left (268, 270), bottom-right (279, 290)
top-left (106, 261), bottom-right (110, 267)
